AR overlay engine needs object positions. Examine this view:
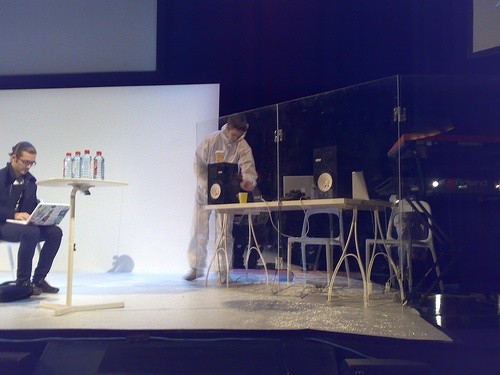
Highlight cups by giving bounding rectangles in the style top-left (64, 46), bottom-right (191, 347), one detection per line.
top-left (215, 150), bottom-right (224, 163)
top-left (238, 192), bottom-right (248, 203)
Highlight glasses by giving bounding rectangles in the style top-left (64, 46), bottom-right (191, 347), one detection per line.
top-left (19, 157), bottom-right (37, 167)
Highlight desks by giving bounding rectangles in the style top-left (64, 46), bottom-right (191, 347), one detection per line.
top-left (35, 177), bottom-right (129, 318)
top-left (198, 198), bottom-right (393, 308)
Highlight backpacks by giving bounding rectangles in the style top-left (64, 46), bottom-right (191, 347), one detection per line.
top-left (0, 281), bottom-right (34, 303)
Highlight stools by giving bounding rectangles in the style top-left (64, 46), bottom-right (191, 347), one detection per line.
top-left (0, 238), bottom-right (42, 284)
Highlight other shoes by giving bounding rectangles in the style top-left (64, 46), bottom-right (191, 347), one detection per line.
top-left (16, 280), bottom-right (42, 295)
top-left (32, 277), bottom-right (60, 293)
top-left (183, 268), bottom-right (206, 280)
top-left (217, 272), bottom-right (232, 284)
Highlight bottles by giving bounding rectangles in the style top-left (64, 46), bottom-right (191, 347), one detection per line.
top-left (63, 152), bottom-right (73, 178)
top-left (93, 151), bottom-right (104, 180)
top-left (81, 150), bottom-right (94, 179)
top-left (71, 151), bottom-right (82, 178)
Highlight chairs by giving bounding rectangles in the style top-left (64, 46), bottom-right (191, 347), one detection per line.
top-left (367, 197), bottom-right (446, 305)
top-left (283, 204), bottom-right (351, 293)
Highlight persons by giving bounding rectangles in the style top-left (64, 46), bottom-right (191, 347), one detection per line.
top-left (184, 113), bottom-right (258, 284)
top-left (0, 142), bottom-right (63, 295)
